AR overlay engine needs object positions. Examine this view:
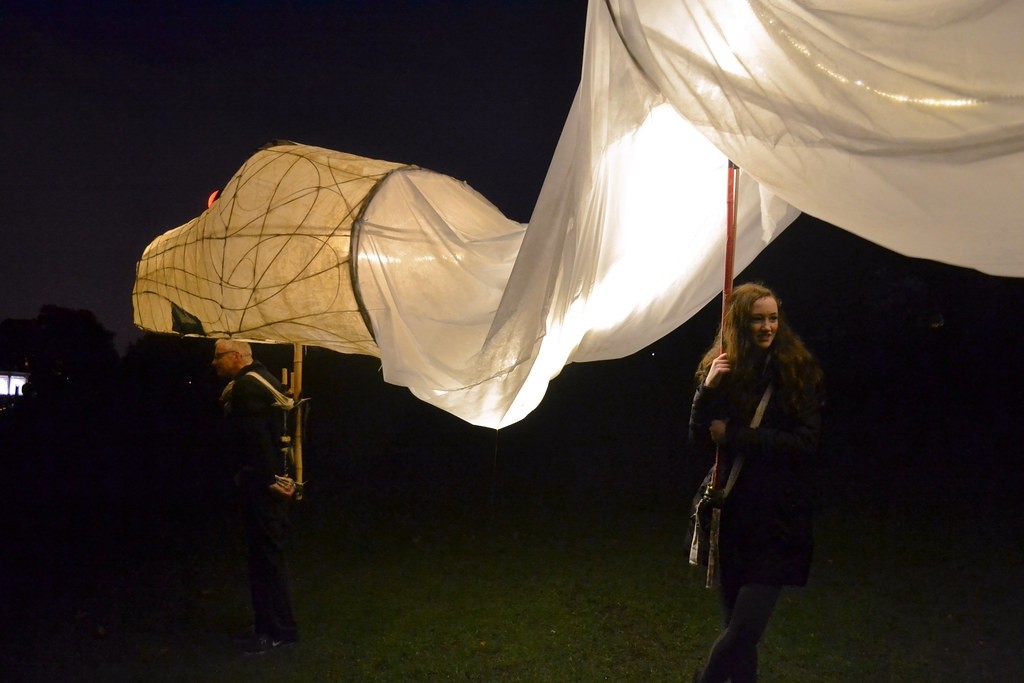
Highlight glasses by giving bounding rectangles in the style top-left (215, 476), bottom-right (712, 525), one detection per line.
top-left (214, 350), bottom-right (238, 359)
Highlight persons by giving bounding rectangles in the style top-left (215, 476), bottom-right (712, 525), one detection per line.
top-left (213, 337), bottom-right (302, 662)
top-left (687, 280), bottom-right (825, 682)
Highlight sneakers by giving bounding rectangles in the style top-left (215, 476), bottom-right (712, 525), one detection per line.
top-left (232, 625), bottom-right (298, 656)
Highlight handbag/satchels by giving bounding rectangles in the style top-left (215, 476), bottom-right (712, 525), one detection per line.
top-left (688, 487), bottom-right (726, 590)
